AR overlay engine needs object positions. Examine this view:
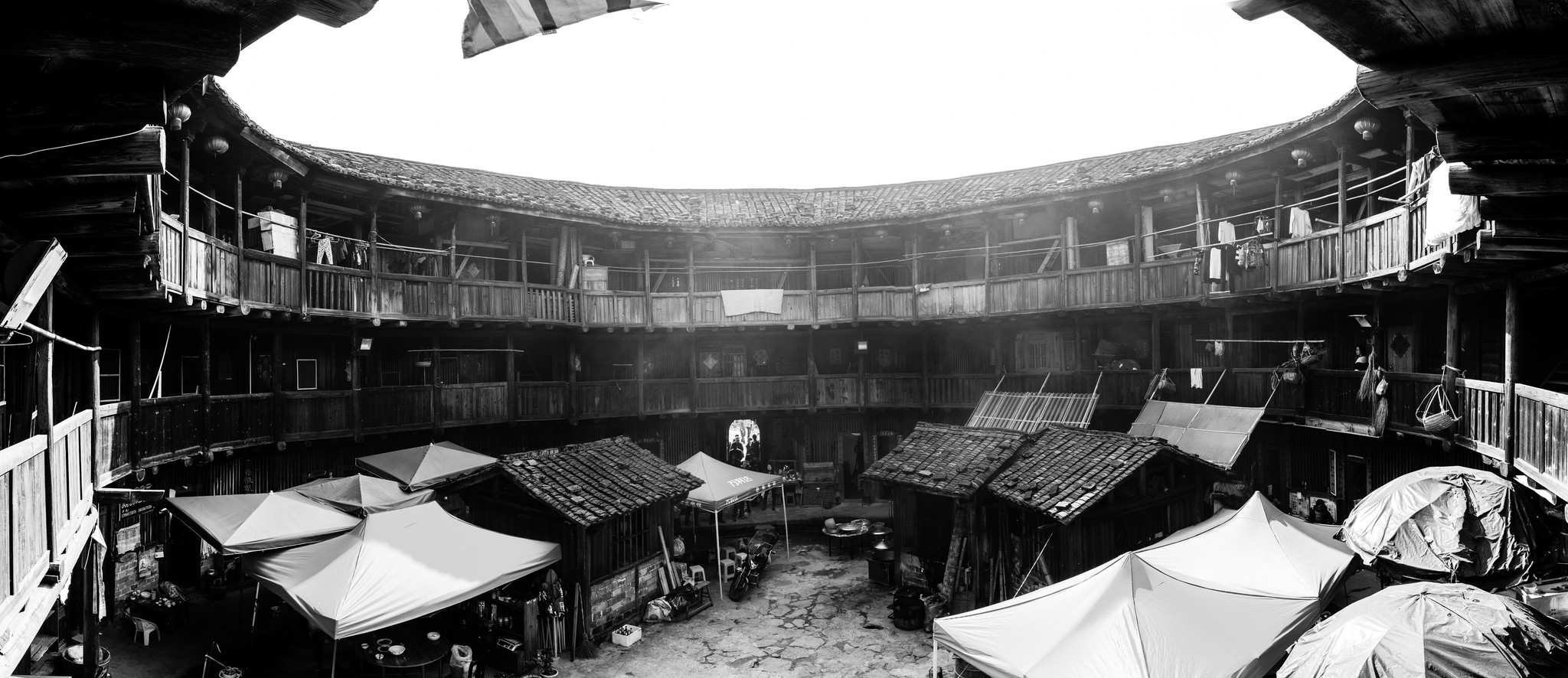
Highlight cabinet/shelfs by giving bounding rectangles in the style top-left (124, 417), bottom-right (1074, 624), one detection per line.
top-left (802, 462), bottom-right (835, 504)
top-left (472, 588), bottom-right (538, 675)
top-left (1516, 589), bottom-right (1568, 625)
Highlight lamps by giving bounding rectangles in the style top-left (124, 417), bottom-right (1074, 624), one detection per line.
top-left (354, 336), bottom-right (374, 356)
top-left (854, 340), bottom-right (868, 354)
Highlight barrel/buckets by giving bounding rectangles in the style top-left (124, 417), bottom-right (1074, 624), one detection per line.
top-left (955, 659), bottom-right (989, 678)
top-left (823, 499), bottom-right (832, 509)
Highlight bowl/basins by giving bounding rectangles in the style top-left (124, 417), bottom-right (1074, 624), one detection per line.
top-left (815, 477), bottom-right (825, 482)
top-left (361, 643), bottom-right (369, 649)
top-left (427, 632), bottom-right (440, 640)
top-left (674, 535), bottom-right (684, 541)
top-left (824, 524), bottom-right (842, 533)
top-left (851, 520), bottom-right (864, 525)
top-left (856, 519), bottom-right (873, 528)
top-left (375, 654), bottom-right (384, 660)
top-left (388, 645), bottom-right (405, 655)
top-left (816, 488), bottom-right (820, 490)
top-left (872, 522), bottom-right (885, 527)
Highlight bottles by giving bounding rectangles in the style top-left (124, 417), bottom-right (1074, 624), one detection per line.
top-left (492, 590), bottom-right (500, 599)
top-left (615, 626), bottom-right (633, 636)
top-left (789, 473), bottom-right (795, 480)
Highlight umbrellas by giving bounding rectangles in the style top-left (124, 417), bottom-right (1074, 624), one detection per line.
top-left (1277, 465), bottom-right (1565, 678)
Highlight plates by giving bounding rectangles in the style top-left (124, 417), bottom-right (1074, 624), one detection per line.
top-left (377, 639), bottom-right (392, 645)
top-left (378, 645), bottom-right (391, 652)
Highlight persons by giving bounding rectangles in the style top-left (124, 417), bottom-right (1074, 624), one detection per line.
top-left (1268, 495), bottom-right (1292, 514)
top-left (685, 419), bottom-right (776, 527)
top-left (1353, 346), bottom-right (1369, 371)
top-left (1308, 499), bottom-right (1334, 525)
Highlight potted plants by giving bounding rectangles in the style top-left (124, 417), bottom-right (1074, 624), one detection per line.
top-left (494, 647), bottom-right (558, 678)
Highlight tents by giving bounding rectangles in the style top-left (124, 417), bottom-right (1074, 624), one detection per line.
top-left (934, 489), bottom-right (1358, 678)
top-left (676, 451), bottom-right (790, 600)
top-left (160, 440), bottom-right (564, 678)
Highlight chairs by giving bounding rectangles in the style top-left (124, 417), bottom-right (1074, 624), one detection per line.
top-left (582, 254), bottom-right (595, 266)
top-left (118, 582), bottom-right (182, 645)
top-left (445, 650), bottom-right (490, 678)
top-left (313, 630), bottom-right (350, 678)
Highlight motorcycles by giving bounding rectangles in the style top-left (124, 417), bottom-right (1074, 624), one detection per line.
top-left (729, 530), bottom-right (779, 602)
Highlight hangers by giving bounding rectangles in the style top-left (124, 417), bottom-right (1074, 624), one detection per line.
top-left (398, 249), bottom-right (433, 259)
top-left (306, 228), bottom-right (370, 251)
top-left (0, 330), bottom-right (33, 346)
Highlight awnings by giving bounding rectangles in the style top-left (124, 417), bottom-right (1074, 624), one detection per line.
top-left (1128, 369), bottom-right (1287, 470)
top-left (965, 371), bottom-right (1103, 432)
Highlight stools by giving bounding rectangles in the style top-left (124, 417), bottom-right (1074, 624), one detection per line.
top-left (688, 546), bottom-right (747, 584)
top-left (855, 473), bottom-right (864, 491)
top-left (786, 492), bottom-right (796, 508)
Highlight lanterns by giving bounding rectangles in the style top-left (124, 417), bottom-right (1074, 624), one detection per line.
top-left (781, 233), bottom-right (795, 245)
top-left (1225, 169), bottom-right (1246, 195)
top-left (663, 234), bottom-right (676, 249)
top-left (1013, 209), bottom-right (1029, 225)
top-left (1159, 184), bottom-right (1175, 202)
top-left (875, 227), bottom-right (889, 240)
top-left (826, 231), bottom-right (839, 245)
top-left (266, 168), bottom-right (288, 189)
top-left (1354, 117), bottom-right (1381, 141)
top-left (1088, 197), bottom-right (1104, 214)
top-left (167, 103), bottom-right (229, 159)
top-left (483, 211), bottom-right (500, 236)
top-left (1291, 145), bottom-right (1314, 168)
top-left (940, 221), bottom-right (954, 236)
top-left (608, 229), bottom-right (621, 249)
top-left (409, 200), bottom-right (427, 219)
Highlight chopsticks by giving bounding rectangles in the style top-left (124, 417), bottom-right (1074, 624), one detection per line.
top-left (357, 644), bottom-right (370, 647)
top-left (379, 652), bottom-right (380, 660)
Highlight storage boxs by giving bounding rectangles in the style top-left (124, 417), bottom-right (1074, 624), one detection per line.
top-left (763, 530), bottom-right (779, 545)
top-left (674, 562), bottom-right (688, 580)
top-left (612, 624), bottom-right (642, 647)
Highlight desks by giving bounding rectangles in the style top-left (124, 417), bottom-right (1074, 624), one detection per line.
top-left (126, 589), bottom-right (185, 635)
top-left (822, 523), bottom-right (868, 560)
top-left (354, 627), bottom-right (449, 678)
